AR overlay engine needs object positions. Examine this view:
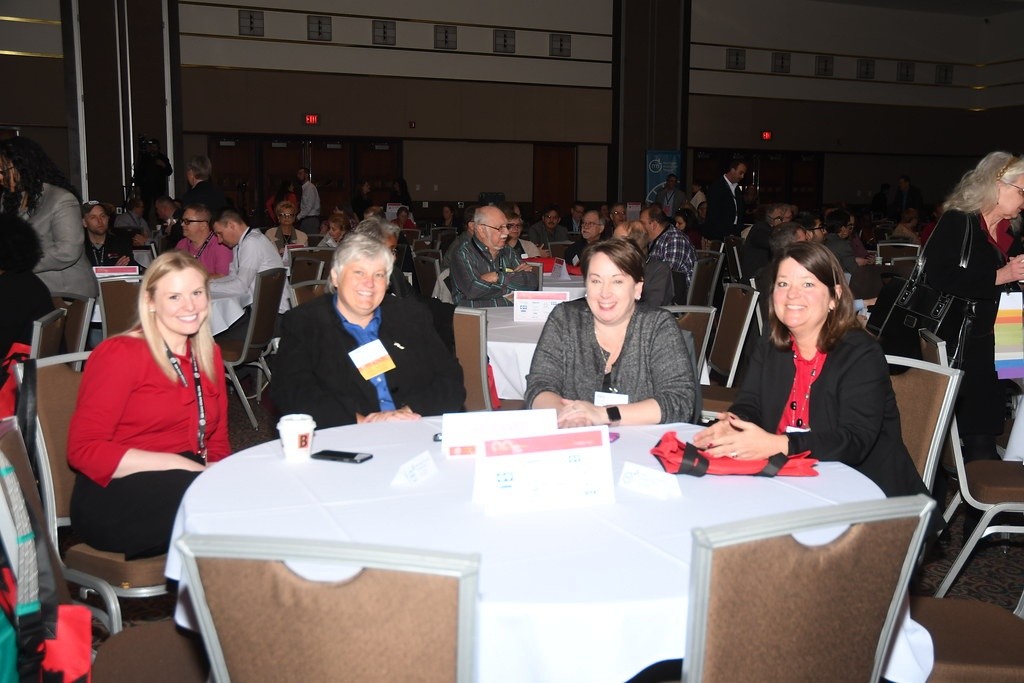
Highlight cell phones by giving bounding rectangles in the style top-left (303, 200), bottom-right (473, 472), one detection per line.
top-left (311, 450), bottom-right (372, 463)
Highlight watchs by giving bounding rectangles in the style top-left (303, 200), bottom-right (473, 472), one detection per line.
top-left (604, 404), bottom-right (622, 427)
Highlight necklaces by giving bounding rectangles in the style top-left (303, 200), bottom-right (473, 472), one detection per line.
top-left (594, 328), bottom-right (608, 373)
top-left (790, 357), bottom-right (818, 428)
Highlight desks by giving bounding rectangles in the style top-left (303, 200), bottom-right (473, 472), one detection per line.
top-left (81, 274), bottom-right (249, 343)
top-left (165, 408), bottom-right (877, 680)
top-left (534, 272), bottom-right (586, 300)
top-left (471, 303), bottom-right (696, 399)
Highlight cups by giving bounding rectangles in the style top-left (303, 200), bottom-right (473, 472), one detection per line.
top-left (277, 414), bottom-right (317, 461)
top-left (156, 225), bottom-right (161, 231)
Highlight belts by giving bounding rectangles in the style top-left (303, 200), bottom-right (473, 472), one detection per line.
top-left (305, 216), bottom-right (318, 218)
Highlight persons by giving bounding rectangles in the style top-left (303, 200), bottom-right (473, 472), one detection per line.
top-left (0, 136), bottom-right (944, 359)
top-left (925, 152), bottom-right (1024, 543)
top-left (67, 247), bottom-right (231, 555)
top-left (525, 238), bottom-right (697, 428)
top-left (269, 232), bottom-right (467, 429)
top-left (694, 240), bottom-right (947, 539)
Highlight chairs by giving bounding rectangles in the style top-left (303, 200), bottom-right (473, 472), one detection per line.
top-left (1, 225), bottom-right (1023, 682)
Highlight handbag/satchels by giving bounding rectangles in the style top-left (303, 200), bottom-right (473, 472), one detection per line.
top-left (871, 207), bottom-right (978, 370)
top-left (650, 431), bottom-right (819, 478)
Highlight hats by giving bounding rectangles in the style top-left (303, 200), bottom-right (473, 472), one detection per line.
top-left (82, 199), bottom-right (106, 218)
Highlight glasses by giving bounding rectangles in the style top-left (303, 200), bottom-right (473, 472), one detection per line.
top-left (810, 223), bottom-right (825, 231)
top-left (0, 166), bottom-right (15, 175)
top-left (277, 213), bottom-right (296, 219)
top-left (1000, 180), bottom-right (1024, 198)
top-left (179, 218), bottom-right (207, 227)
top-left (477, 222), bottom-right (506, 233)
top-left (544, 215), bottom-right (559, 220)
top-left (506, 223), bottom-right (521, 229)
top-left (611, 211), bottom-right (626, 216)
top-left (581, 221), bottom-right (601, 228)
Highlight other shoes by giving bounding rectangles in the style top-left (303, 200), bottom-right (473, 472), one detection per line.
top-left (864, 326), bottom-right (879, 339)
top-left (961, 526), bottom-right (991, 549)
top-left (934, 527), bottom-right (951, 549)
